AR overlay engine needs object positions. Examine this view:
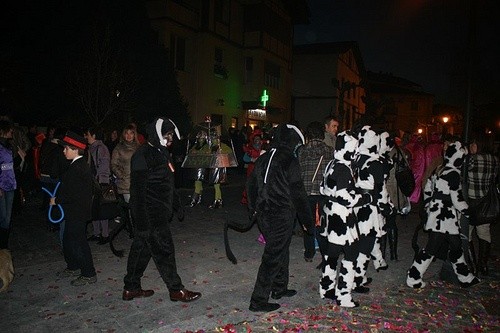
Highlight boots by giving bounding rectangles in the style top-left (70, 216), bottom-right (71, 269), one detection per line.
top-left (0, 228), bottom-right (9, 249)
top-left (388, 228), bottom-right (398, 261)
top-left (477, 238), bottom-right (491, 276)
top-left (380, 233), bottom-right (387, 259)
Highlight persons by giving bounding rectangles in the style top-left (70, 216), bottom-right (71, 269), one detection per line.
top-left (352, 125), bottom-right (389, 285)
top-left (406, 141), bottom-right (481, 289)
top-left (111, 121), bottom-right (140, 259)
top-left (0, 117), bottom-right (500, 312)
top-left (49, 129), bottom-right (97, 286)
top-left (122, 117), bottom-right (202, 302)
top-left (465, 135), bottom-right (500, 277)
top-left (0, 117), bottom-right (18, 260)
top-left (319, 130), bottom-right (361, 309)
top-left (182, 117), bottom-right (238, 208)
top-left (296, 121), bottom-right (335, 263)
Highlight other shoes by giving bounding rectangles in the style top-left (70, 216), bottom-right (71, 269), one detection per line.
top-left (184, 194), bottom-right (204, 208)
top-left (97, 237), bottom-right (110, 245)
top-left (304, 256), bottom-right (313, 262)
top-left (207, 199), bottom-right (224, 210)
top-left (87, 235), bottom-right (100, 241)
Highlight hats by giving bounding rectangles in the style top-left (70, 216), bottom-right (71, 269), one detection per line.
top-left (57, 132), bottom-right (87, 150)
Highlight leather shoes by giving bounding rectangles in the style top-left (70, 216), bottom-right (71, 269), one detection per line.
top-left (249, 303), bottom-right (280, 312)
top-left (123, 289), bottom-right (154, 301)
top-left (169, 288), bottom-right (201, 302)
top-left (271, 290), bottom-right (297, 300)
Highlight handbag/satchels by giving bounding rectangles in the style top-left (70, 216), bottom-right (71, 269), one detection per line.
top-left (101, 186), bottom-right (116, 203)
top-left (395, 148), bottom-right (415, 197)
top-left (468, 183), bottom-right (500, 226)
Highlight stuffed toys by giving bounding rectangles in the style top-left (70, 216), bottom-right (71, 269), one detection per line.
top-left (223, 123), bottom-right (310, 311)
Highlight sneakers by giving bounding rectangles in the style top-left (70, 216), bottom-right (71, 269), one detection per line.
top-left (56, 269), bottom-right (81, 278)
top-left (71, 275), bottom-right (97, 287)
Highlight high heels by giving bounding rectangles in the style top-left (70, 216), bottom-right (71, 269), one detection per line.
top-left (377, 265), bottom-right (388, 272)
